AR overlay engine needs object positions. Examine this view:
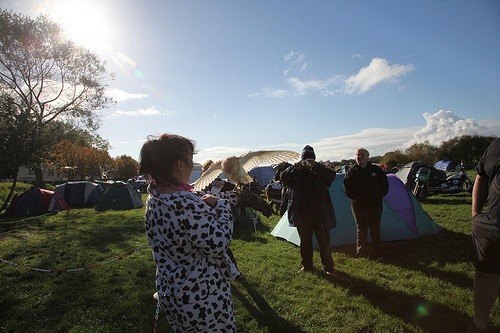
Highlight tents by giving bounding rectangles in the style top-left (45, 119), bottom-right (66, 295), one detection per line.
top-left (269, 173), bottom-right (446, 247)
top-left (95, 181), bottom-right (145, 211)
top-left (54, 181), bottom-right (104, 208)
top-left (189, 164), bottom-right (276, 190)
top-left (2, 185), bottom-right (70, 219)
top-left (433, 159), bottom-right (466, 172)
top-left (394, 161), bottom-right (448, 191)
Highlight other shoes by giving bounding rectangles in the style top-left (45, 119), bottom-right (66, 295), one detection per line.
top-left (297, 265), bottom-right (311, 274)
top-left (322, 264), bottom-right (336, 276)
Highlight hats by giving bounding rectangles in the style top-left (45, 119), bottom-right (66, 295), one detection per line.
top-left (301, 144), bottom-right (315, 160)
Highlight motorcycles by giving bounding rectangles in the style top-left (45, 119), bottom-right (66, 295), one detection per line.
top-left (413, 160), bottom-right (474, 200)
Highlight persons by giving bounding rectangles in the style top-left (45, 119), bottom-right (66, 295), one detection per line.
top-left (281, 145), bottom-right (336, 275)
top-left (466, 137), bottom-right (500, 333)
top-left (343, 148), bottom-right (389, 258)
top-left (139, 134), bottom-right (272, 333)
top-left (272, 161), bottom-right (293, 218)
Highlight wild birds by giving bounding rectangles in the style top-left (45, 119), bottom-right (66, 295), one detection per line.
top-left (187, 149), bottom-right (301, 194)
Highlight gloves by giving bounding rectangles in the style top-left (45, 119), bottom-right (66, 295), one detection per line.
top-left (236, 188), bottom-right (273, 218)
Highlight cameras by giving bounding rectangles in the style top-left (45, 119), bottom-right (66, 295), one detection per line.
top-left (302, 160), bottom-right (310, 167)
